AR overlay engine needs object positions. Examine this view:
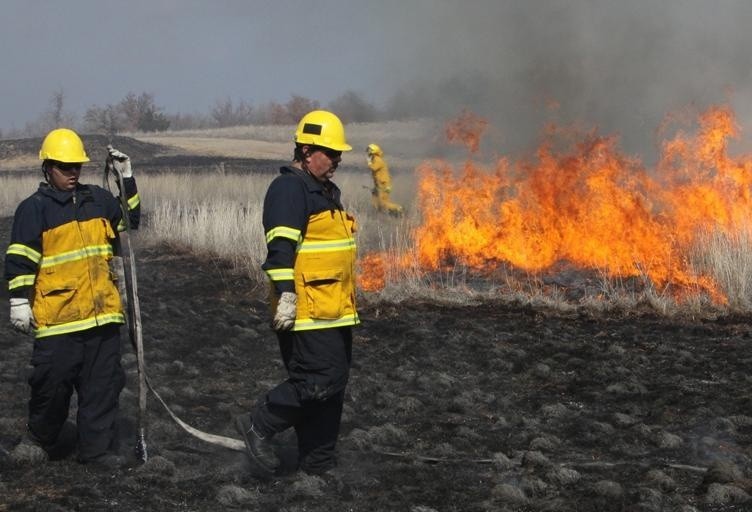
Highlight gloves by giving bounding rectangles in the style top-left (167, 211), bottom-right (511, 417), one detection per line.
top-left (8, 298), bottom-right (39, 333)
top-left (270, 292), bottom-right (299, 334)
top-left (106, 145), bottom-right (132, 181)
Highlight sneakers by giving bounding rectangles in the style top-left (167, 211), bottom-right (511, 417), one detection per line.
top-left (84, 454), bottom-right (127, 470)
top-left (298, 454), bottom-right (363, 482)
top-left (21, 432), bottom-right (53, 461)
top-left (235, 413), bottom-right (280, 476)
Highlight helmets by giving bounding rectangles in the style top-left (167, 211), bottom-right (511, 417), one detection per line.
top-left (294, 110), bottom-right (352, 152)
top-left (38, 128), bottom-right (90, 163)
top-left (368, 143), bottom-right (383, 156)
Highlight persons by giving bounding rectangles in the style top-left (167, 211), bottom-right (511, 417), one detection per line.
top-left (233, 111), bottom-right (360, 484)
top-left (5, 127), bottom-right (140, 468)
top-left (364, 143), bottom-right (405, 216)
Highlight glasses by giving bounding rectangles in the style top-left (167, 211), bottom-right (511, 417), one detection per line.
top-left (316, 147), bottom-right (342, 159)
top-left (51, 163), bottom-right (82, 171)
